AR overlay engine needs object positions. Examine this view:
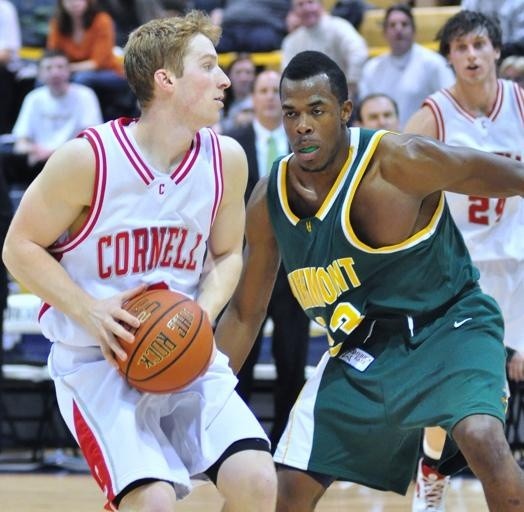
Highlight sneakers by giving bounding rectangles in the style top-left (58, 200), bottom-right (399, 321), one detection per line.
top-left (411, 456), bottom-right (451, 512)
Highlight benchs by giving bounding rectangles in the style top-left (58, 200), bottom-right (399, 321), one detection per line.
top-left (0, 294), bottom-right (330, 474)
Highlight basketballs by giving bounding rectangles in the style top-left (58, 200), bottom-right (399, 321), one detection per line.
top-left (112, 290), bottom-right (213, 392)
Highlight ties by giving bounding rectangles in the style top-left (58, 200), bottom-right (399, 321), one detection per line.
top-left (264, 135), bottom-right (279, 175)
